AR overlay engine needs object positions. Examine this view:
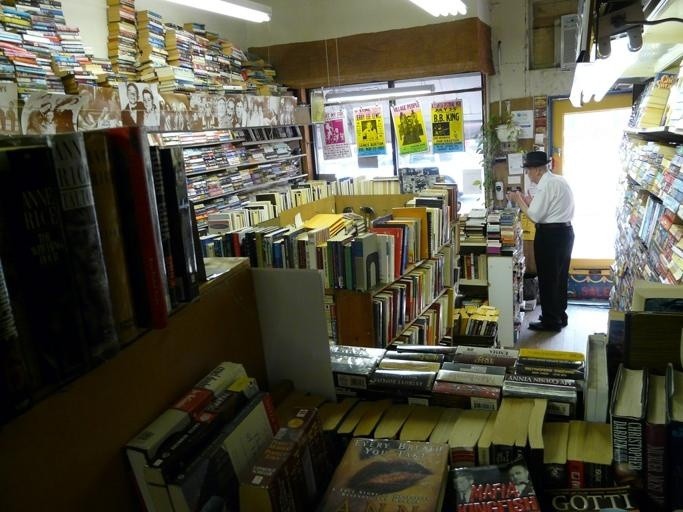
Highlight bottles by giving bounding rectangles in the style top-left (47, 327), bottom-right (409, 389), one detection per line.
top-left (495, 179), bottom-right (504, 200)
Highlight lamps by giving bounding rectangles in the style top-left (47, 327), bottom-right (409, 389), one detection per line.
top-left (569, 1), bottom-right (644, 108)
top-left (169, 0), bottom-right (273, 23)
top-left (409, 0), bottom-right (468, 19)
top-left (325, 34), bottom-right (437, 106)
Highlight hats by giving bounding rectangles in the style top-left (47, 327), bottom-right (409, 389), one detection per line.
top-left (520, 150), bottom-right (551, 168)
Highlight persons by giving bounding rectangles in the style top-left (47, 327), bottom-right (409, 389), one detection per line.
top-left (506, 151), bottom-right (574, 331)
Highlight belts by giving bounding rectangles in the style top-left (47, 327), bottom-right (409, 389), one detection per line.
top-left (535, 222), bottom-right (571, 228)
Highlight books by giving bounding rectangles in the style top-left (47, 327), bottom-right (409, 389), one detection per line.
top-left (149, 127), bottom-right (307, 235)
top-left (128, 278), bottom-right (683, 512)
top-left (608, 57), bottom-right (682, 312)
top-left (1, 126), bottom-right (206, 413)
top-left (1, 1), bottom-right (300, 135)
top-left (209, 166), bottom-right (524, 343)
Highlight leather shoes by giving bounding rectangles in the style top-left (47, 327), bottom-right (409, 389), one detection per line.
top-left (539, 313), bottom-right (568, 326)
top-left (528, 320), bottom-right (563, 332)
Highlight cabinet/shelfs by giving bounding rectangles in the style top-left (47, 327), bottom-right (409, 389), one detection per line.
top-left (336, 240), bottom-right (459, 345)
top-left (148, 124), bottom-right (314, 237)
top-left (608, 52), bottom-right (683, 311)
top-left (457, 209), bottom-right (527, 347)
